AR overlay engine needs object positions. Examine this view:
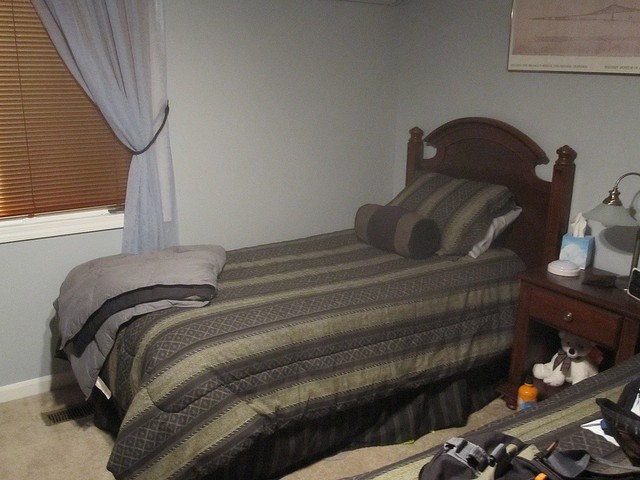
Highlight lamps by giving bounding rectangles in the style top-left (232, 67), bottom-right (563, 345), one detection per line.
top-left (582, 172), bottom-right (640, 292)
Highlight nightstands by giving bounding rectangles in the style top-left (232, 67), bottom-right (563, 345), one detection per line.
top-left (507, 265), bottom-right (640, 410)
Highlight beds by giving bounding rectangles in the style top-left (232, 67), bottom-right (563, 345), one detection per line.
top-left (53, 117), bottom-right (578, 480)
top-left (339, 355), bottom-right (640, 479)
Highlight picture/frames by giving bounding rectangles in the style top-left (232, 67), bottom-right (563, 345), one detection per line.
top-left (507, 0), bottom-right (640, 76)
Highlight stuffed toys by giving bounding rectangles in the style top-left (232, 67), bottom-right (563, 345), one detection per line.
top-left (532, 330), bottom-right (600, 388)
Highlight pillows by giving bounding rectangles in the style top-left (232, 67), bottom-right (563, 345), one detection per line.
top-left (354, 203), bottom-right (440, 260)
top-left (385, 171), bottom-right (516, 261)
top-left (468, 205), bottom-right (522, 258)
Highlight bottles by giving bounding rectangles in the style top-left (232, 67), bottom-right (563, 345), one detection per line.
top-left (517, 377), bottom-right (540, 412)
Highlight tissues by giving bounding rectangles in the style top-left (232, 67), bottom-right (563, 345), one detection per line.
top-left (560, 214), bottom-right (595, 269)
